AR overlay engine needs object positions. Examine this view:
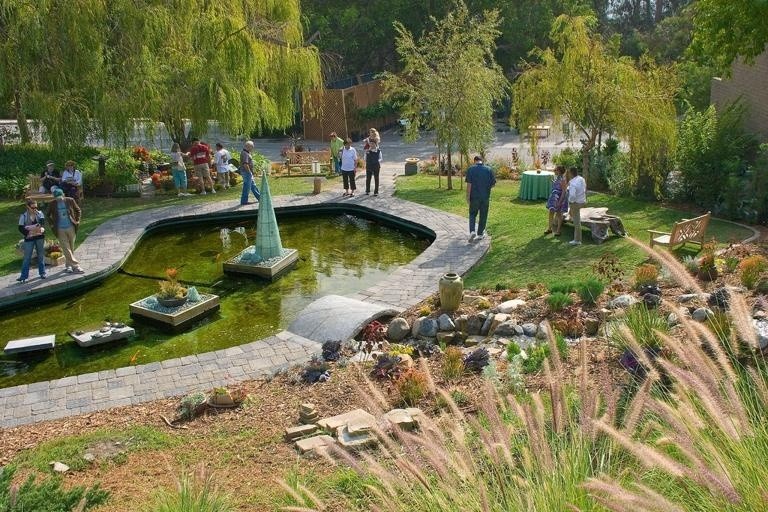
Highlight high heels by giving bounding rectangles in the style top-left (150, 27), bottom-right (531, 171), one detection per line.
top-left (41, 273), bottom-right (46, 279)
top-left (16, 276), bottom-right (25, 283)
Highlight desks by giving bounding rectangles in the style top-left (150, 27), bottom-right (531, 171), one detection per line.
top-left (518, 170), bottom-right (555, 200)
top-left (527, 124), bottom-right (551, 138)
top-left (25, 195), bottom-right (64, 205)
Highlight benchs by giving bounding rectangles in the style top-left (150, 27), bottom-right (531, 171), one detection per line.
top-left (21, 175), bottom-right (85, 203)
top-left (285, 148), bottom-right (333, 175)
top-left (647, 210), bottom-right (713, 254)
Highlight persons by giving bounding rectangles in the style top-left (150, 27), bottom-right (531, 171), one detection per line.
top-left (567, 167), bottom-right (586, 244)
top-left (465, 156), bottom-right (496, 243)
top-left (364, 129), bottom-right (380, 151)
top-left (45, 189), bottom-right (86, 274)
top-left (17, 201), bottom-right (48, 281)
top-left (329, 131), bottom-right (345, 175)
top-left (40, 159), bottom-right (62, 207)
top-left (544, 165), bottom-right (569, 236)
top-left (170, 136), bottom-right (260, 206)
top-left (337, 138), bottom-right (359, 197)
top-left (61, 160), bottom-right (84, 208)
top-left (364, 137), bottom-right (383, 196)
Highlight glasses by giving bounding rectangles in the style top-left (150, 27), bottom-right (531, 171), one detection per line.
top-left (29, 205), bottom-right (37, 209)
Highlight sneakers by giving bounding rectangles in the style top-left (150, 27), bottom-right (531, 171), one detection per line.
top-left (568, 239), bottom-right (582, 246)
top-left (177, 192), bottom-right (192, 198)
top-left (73, 265), bottom-right (86, 274)
top-left (65, 267), bottom-right (73, 273)
top-left (477, 232), bottom-right (485, 240)
top-left (468, 231), bottom-right (476, 243)
top-left (343, 192), bottom-right (380, 197)
top-left (200, 191), bottom-right (207, 195)
top-left (212, 188), bottom-right (217, 193)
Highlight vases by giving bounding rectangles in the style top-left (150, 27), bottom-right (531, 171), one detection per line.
top-left (157, 296), bottom-right (185, 307)
top-left (438, 272), bottom-right (463, 311)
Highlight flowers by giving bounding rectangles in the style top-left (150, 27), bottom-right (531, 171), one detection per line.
top-left (157, 261), bottom-right (187, 300)
top-left (45, 244), bottom-right (64, 258)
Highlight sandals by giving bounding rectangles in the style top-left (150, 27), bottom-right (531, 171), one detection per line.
top-left (544, 229), bottom-right (552, 234)
top-left (555, 231), bottom-right (561, 236)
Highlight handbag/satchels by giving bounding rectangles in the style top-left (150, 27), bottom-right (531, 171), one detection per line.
top-left (339, 158), bottom-right (342, 167)
top-left (177, 160), bottom-right (187, 171)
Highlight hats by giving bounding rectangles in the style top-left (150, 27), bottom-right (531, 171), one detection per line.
top-left (46, 160), bottom-right (54, 167)
top-left (53, 188), bottom-right (64, 197)
